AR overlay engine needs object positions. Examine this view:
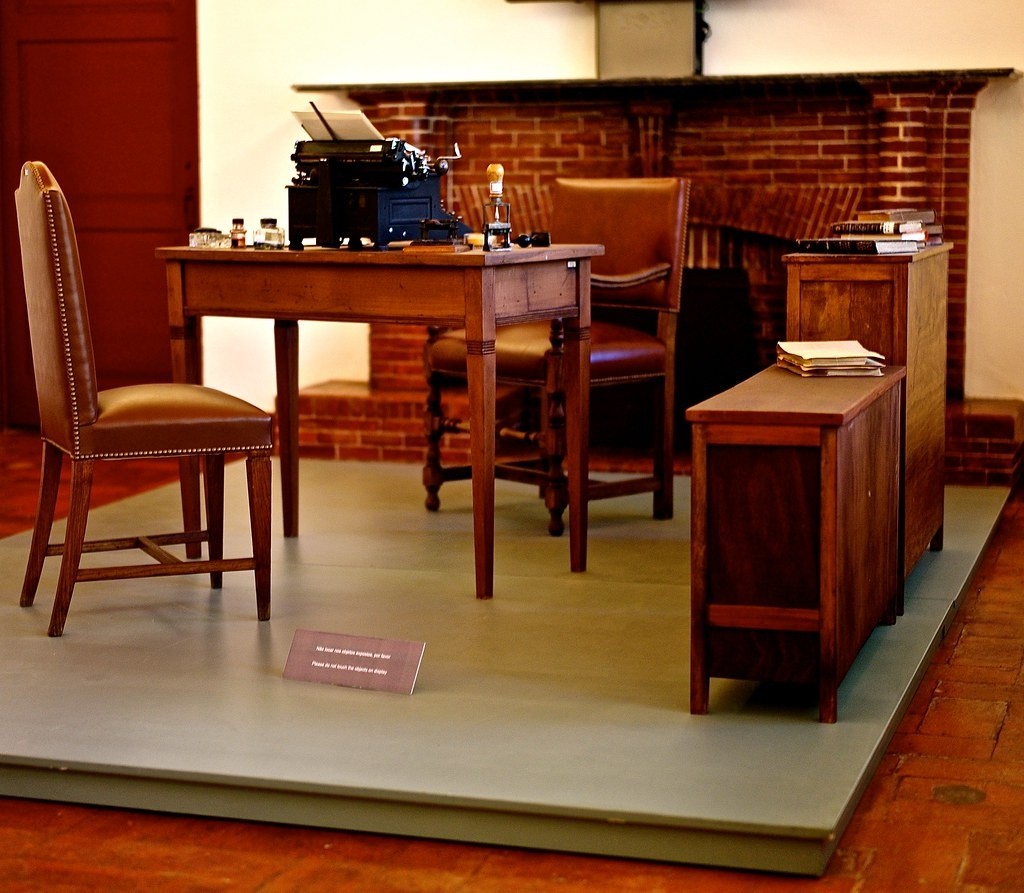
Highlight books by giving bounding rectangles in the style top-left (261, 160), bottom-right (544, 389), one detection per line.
top-left (777, 338), bottom-right (886, 379)
top-left (797, 205), bottom-right (943, 255)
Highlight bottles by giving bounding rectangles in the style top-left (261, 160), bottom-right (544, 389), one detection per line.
top-left (230, 218), bottom-right (247, 248)
top-left (253, 219), bottom-right (286, 250)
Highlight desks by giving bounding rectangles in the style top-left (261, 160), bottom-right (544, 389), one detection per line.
top-left (156, 244), bottom-right (606, 600)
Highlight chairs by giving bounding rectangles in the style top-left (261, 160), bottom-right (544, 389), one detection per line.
top-left (14, 159), bottom-right (277, 637)
top-left (424, 172), bottom-right (693, 541)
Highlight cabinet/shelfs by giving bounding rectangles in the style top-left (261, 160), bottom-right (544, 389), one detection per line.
top-left (684, 364), bottom-right (906, 724)
top-left (782, 240), bottom-right (956, 603)
